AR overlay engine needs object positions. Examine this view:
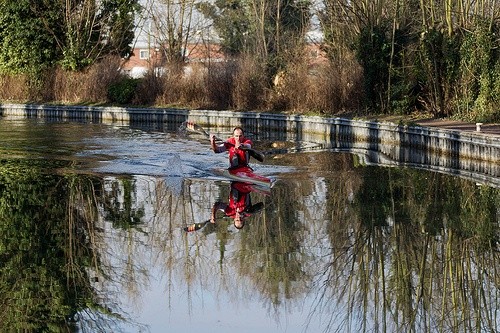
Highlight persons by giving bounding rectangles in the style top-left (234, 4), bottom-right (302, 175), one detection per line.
top-left (188, 181), bottom-right (270, 232)
top-left (210, 127), bottom-right (277, 183)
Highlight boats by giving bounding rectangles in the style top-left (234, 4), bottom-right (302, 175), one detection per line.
top-left (206, 166), bottom-right (272, 187)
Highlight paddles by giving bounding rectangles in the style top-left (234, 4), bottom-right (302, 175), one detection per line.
top-left (182, 121), bottom-right (266, 163)
top-left (183, 200), bottom-right (265, 232)
